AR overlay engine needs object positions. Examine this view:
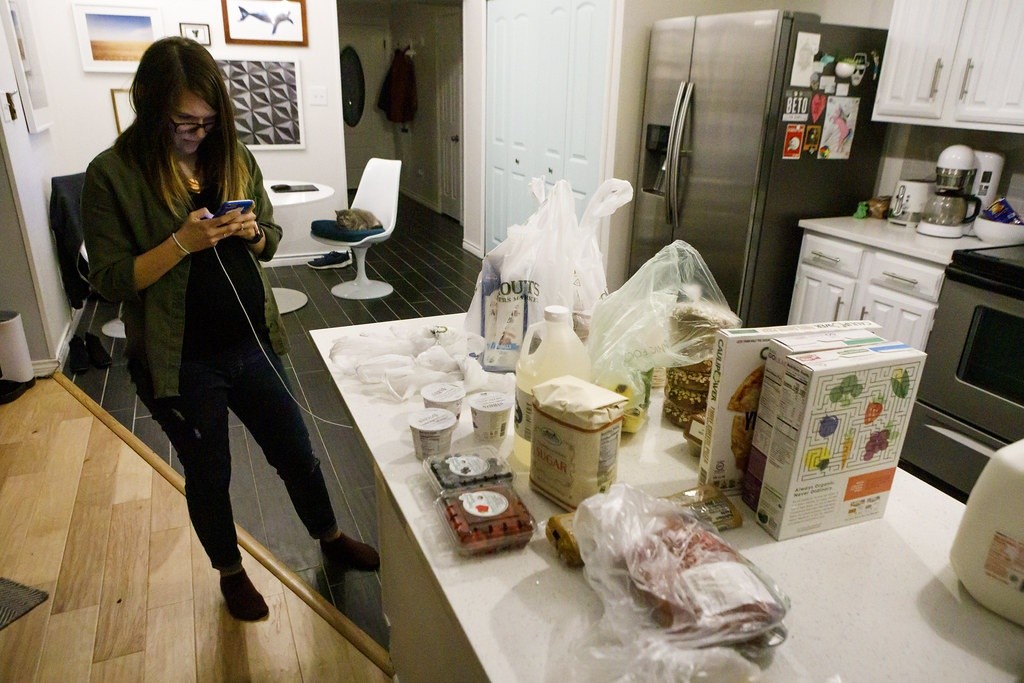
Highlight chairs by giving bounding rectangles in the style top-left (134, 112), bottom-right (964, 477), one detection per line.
top-left (52, 172), bottom-right (128, 339)
top-left (310, 158), bottom-right (402, 300)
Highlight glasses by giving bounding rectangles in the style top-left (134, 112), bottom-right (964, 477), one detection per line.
top-left (168, 115), bottom-right (221, 134)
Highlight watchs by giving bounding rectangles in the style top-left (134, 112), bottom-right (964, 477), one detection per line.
top-left (245, 221), bottom-right (263, 244)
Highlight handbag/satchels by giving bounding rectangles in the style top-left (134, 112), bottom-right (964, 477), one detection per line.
top-left (462, 174), bottom-right (634, 373)
top-left (572, 482), bottom-right (792, 683)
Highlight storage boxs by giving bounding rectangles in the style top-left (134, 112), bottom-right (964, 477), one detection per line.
top-left (698, 319), bottom-right (927, 540)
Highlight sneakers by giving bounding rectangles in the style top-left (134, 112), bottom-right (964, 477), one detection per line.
top-left (306, 250), bottom-right (353, 270)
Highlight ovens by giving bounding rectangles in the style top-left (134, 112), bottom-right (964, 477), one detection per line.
top-left (899, 268), bottom-right (1024, 496)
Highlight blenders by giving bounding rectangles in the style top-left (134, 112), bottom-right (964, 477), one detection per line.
top-left (915, 143), bottom-right (981, 238)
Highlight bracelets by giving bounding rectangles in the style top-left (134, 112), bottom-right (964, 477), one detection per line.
top-left (172, 233), bottom-right (190, 254)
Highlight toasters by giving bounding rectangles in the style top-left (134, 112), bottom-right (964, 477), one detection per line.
top-left (887, 178), bottom-right (936, 229)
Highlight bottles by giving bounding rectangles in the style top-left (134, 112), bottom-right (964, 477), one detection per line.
top-left (511, 304), bottom-right (593, 463)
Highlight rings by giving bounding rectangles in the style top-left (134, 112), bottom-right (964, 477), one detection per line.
top-left (240, 224), bottom-right (244, 231)
top-left (223, 234), bottom-right (226, 238)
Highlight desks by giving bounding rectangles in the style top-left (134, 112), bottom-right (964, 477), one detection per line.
top-left (309, 311), bottom-right (1024, 683)
top-left (258, 179), bottom-right (335, 316)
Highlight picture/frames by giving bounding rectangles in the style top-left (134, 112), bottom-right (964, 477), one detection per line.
top-left (180, 23), bottom-right (211, 46)
top-left (221, 0), bottom-right (308, 47)
top-left (0, 0), bottom-right (56, 135)
top-left (70, 5), bottom-right (155, 74)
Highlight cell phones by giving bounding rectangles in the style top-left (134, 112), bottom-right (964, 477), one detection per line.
top-left (212, 200), bottom-right (254, 218)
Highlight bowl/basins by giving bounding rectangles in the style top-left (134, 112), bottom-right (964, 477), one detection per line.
top-left (973, 215), bottom-right (1024, 246)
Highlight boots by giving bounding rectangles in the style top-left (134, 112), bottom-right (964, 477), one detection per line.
top-left (84, 333), bottom-right (114, 369)
top-left (68, 334), bottom-right (91, 373)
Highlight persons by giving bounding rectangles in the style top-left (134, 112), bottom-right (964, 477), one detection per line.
top-left (78, 37), bottom-right (379, 619)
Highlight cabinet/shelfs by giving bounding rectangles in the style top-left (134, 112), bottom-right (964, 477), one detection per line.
top-left (484, 0), bottom-right (616, 267)
top-left (870, 0), bottom-right (1024, 136)
top-left (787, 230), bottom-right (947, 354)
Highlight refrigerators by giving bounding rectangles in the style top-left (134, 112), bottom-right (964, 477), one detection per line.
top-left (625, 7), bottom-right (888, 321)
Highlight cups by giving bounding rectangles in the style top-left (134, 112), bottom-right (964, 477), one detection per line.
top-left (409, 407), bottom-right (457, 461)
top-left (421, 382), bottom-right (467, 421)
top-left (468, 391), bottom-right (513, 442)
